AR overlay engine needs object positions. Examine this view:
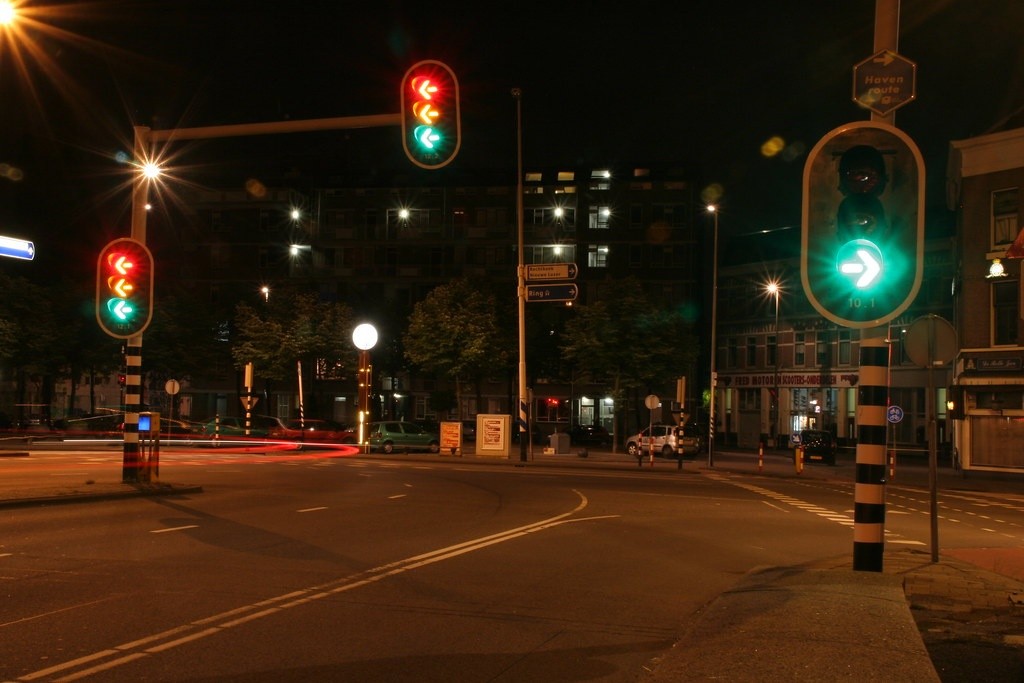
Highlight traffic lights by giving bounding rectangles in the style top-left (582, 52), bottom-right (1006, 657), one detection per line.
top-left (546, 398), bottom-right (559, 406)
top-left (398, 60), bottom-right (463, 171)
top-left (800, 122), bottom-right (925, 337)
top-left (95, 236), bottom-right (153, 340)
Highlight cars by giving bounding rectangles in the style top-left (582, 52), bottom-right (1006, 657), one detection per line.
top-left (369, 421), bottom-right (440, 455)
top-left (190, 416), bottom-right (267, 437)
top-left (793, 429), bottom-right (837, 466)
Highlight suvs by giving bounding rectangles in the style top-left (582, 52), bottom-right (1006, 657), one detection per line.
top-left (624, 425), bottom-right (700, 460)
top-left (270, 418), bottom-right (356, 444)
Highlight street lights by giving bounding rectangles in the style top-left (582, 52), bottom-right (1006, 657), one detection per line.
top-left (706, 203), bottom-right (718, 468)
top-left (763, 280), bottom-right (780, 454)
top-left (510, 86), bottom-right (529, 460)
top-left (352, 324), bottom-right (379, 457)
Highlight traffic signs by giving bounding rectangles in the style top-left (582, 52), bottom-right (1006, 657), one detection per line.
top-left (853, 49), bottom-right (916, 119)
top-left (522, 263), bottom-right (580, 282)
top-left (524, 284), bottom-right (578, 304)
